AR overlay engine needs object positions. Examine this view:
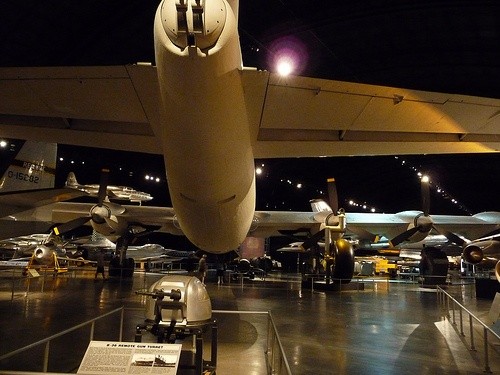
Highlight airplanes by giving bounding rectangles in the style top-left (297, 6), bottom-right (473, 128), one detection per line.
top-left (1, 0), bottom-right (499, 271)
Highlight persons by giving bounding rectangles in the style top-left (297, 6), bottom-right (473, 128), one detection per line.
top-left (94, 257), bottom-right (106, 280)
top-left (185, 251), bottom-right (197, 276)
top-left (197, 255), bottom-right (208, 283)
